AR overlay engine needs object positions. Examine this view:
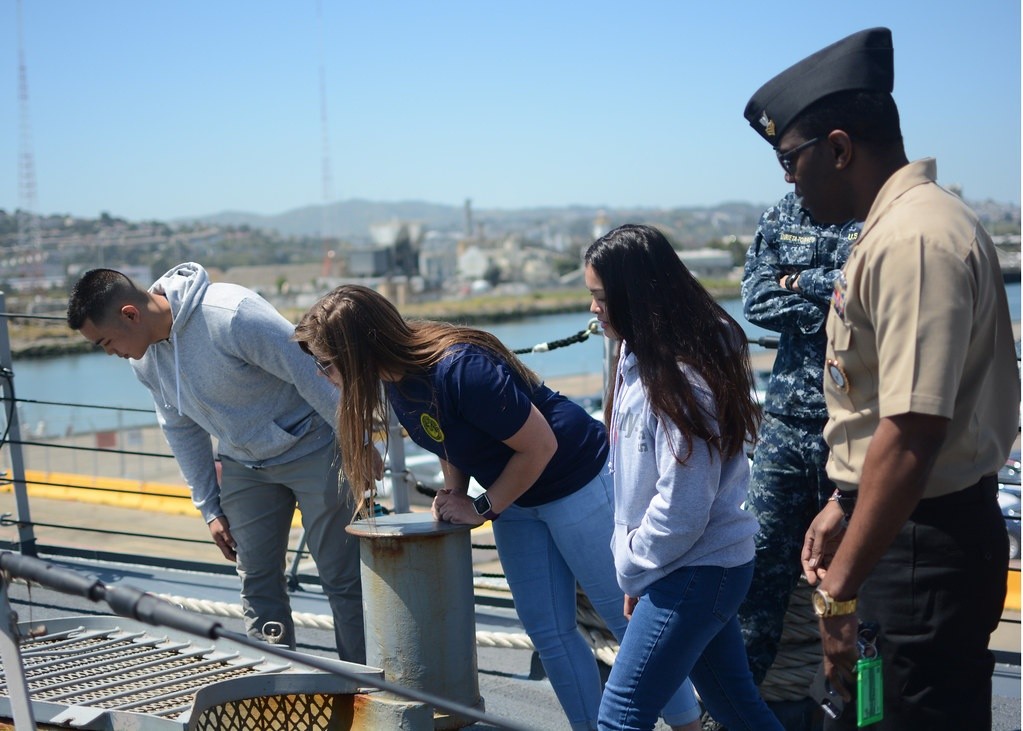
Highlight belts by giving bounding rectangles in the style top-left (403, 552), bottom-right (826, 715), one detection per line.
top-left (838, 475), bottom-right (999, 520)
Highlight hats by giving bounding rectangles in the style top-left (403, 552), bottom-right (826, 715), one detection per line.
top-left (744, 27), bottom-right (894, 147)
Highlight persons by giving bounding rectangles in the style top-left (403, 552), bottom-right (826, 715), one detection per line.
top-left (742, 27), bottom-right (1021, 731)
top-left (66, 259), bottom-right (385, 667)
top-left (295, 284), bottom-right (704, 731)
top-left (705, 188), bottom-right (867, 731)
top-left (583, 225), bottom-right (784, 731)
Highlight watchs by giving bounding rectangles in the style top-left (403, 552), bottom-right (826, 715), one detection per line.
top-left (809, 589), bottom-right (860, 619)
top-left (471, 493), bottom-right (500, 522)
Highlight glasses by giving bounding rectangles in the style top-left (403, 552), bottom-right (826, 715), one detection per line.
top-left (312, 353), bottom-right (336, 377)
top-left (776, 137), bottom-right (822, 174)
